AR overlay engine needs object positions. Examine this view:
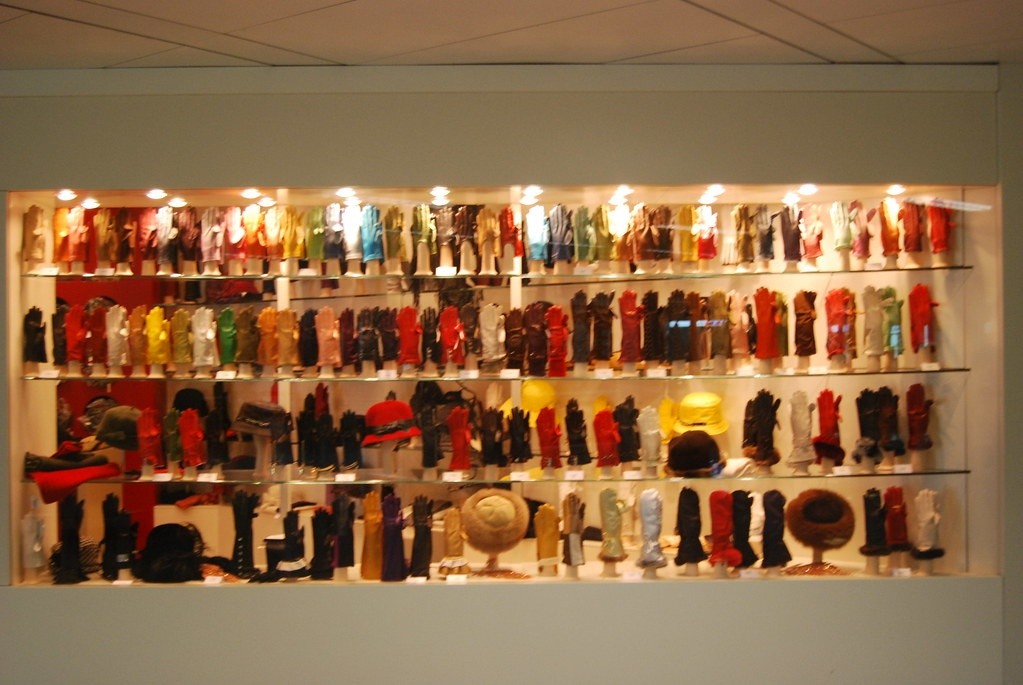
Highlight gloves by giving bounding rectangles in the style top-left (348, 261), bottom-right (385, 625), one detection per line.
top-left (228, 489), bottom-right (472, 581)
top-left (858, 485), bottom-right (944, 557)
top-left (142, 408), bottom-right (229, 469)
top-left (534, 489), bottom-right (792, 570)
top-left (20, 438), bottom-right (120, 503)
top-left (22, 197), bottom-right (954, 263)
top-left (24, 284), bottom-right (939, 375)
top-left (51, 493), bottom-right (130, 583)
top-left (293, 384), bottom-right (364, 471)
top-left (419, 395), bottom-right (643, 472)
top-left (742, 382), bottom-right (934, 464)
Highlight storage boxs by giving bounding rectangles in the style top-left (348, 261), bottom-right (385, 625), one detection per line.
top-left (361, 448), bottom-right (453, 510)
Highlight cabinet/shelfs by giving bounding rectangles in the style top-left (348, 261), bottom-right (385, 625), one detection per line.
top-left (20, 269), bottom-right (972, 487)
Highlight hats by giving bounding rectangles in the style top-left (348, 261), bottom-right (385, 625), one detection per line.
top-left (359, 400), bottom-right (421, 447)
top-left (231, 401), bottom-right (289, 440)
top-left (133, 523), bottom-right (204, 582)
top-left (672, 392), bottom-right (727, 436)
top-left (787, 489), bottom-right (857, 550)
top-left (96, 405), bottom-right (140, 449)
top-left (663, 431), bottom-right (725, 476)
top-left (461, 487), bottom-right (530, 553)
